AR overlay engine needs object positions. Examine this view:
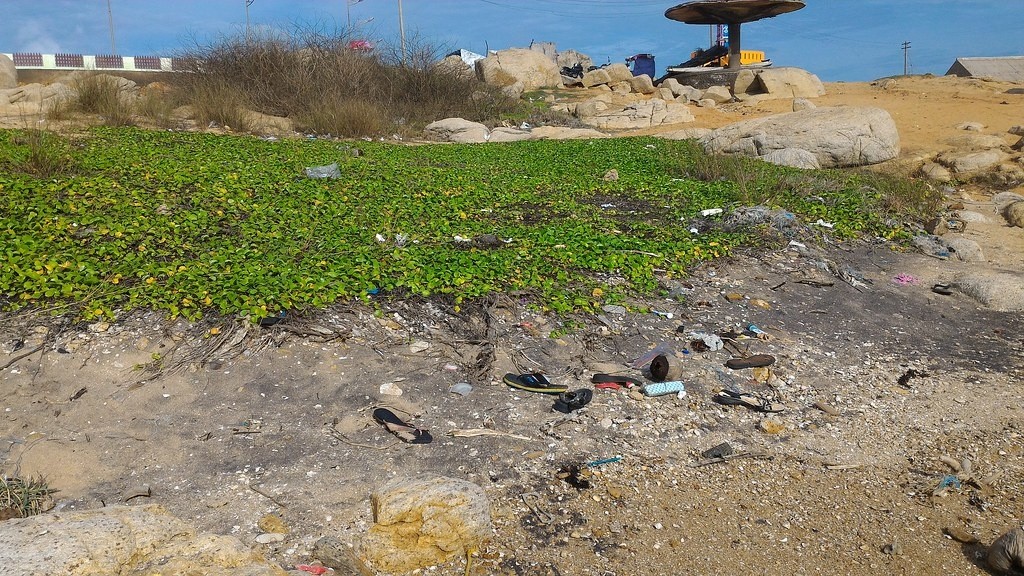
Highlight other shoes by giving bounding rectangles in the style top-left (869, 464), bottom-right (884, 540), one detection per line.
top-left (552, 388), bottom-right (592, 415)
top-left (727, 355), bottom-right (776, 369)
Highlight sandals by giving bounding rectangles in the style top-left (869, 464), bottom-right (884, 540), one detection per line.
top-left (592, 374), bottom-right (642, 391)
top-left (718, 389), bottom-right (785, 412)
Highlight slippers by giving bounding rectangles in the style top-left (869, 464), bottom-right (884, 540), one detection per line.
top-left (932, 284), bottom-right (954, 294)
top-left (374, 408), bottom-right (433, 444)
top-left (504, 372), bottom-right (568, 393)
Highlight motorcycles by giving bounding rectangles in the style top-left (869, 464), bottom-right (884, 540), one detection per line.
top-left (560, 61), bottom-right (584, 79)
top-left (588, 54), bottom-right (611, 72)
